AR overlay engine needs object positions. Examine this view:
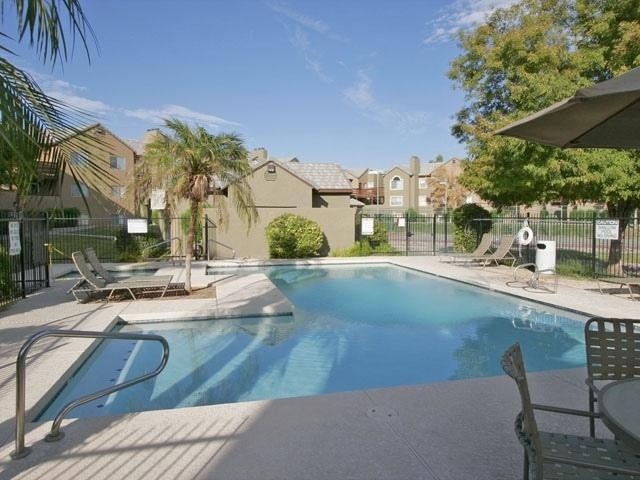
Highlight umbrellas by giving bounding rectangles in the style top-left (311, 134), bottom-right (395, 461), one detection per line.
top-left (493, 66), bottom-right (640, 151)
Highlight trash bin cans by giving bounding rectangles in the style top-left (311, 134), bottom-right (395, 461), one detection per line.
top-left (536, 240), bottom-right (556, 274)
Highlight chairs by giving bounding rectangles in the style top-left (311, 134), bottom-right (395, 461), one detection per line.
top-left (498, 317), bottom-right (640, 480)
top-left (442, 232), bottom-right (516, 271)
top-left (63, 244), bottom-right (174, 302)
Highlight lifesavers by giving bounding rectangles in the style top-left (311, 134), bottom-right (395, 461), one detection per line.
top-left (517, 228), bottom-right (533, 247)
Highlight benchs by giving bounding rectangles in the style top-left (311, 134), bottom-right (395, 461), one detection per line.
top-left (594, 274), bottom-right (640, 300)
